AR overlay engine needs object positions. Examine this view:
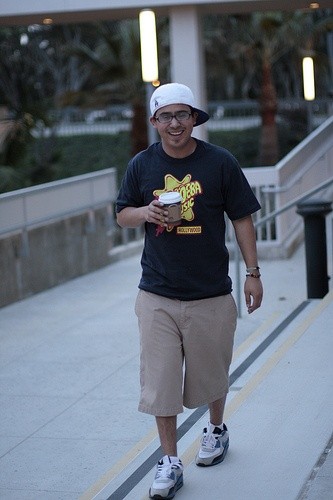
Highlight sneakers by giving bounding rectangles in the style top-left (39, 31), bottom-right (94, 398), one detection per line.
top-left (196, 423), bottom-right (229, 466)
top-left (149, 454), bottom-right (183, 500)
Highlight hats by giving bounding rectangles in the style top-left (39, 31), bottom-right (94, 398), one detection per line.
top-left (149, 83), bottom-right (209, 127)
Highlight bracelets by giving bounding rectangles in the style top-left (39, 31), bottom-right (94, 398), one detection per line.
top-left (245, 266), bottom-right (260, 270)
top-left (246, 273), bottom-right (261, 278)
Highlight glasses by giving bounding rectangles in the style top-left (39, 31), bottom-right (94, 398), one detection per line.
top-left (154, 110), bottom-right (194, 124)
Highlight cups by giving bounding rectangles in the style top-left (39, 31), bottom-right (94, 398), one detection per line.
top-left (158, 192), bottom-right (183, 226)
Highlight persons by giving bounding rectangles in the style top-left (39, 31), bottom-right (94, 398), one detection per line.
top-left (113, 83), bottom-right (263, 500)
